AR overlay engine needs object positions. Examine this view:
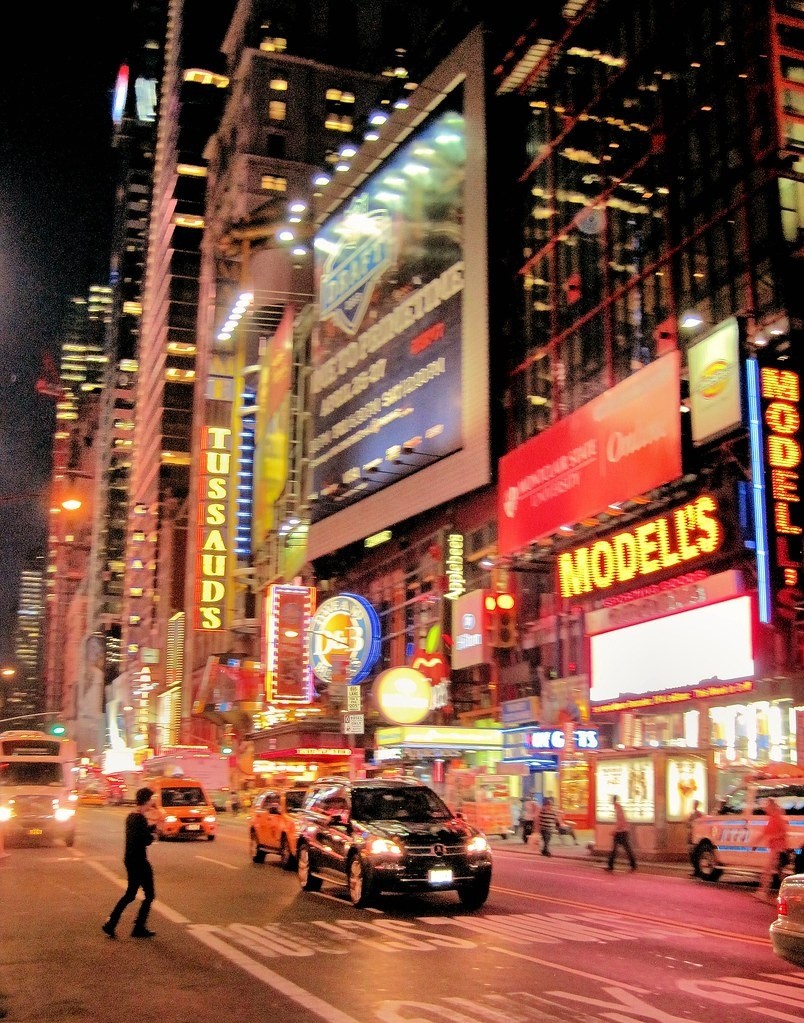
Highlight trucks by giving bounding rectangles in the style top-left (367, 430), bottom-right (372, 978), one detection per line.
top-left (144, 752), bottom-right (232, 810)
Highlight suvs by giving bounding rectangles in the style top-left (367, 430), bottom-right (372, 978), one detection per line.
top-left (248, 787), bottom-right (307, 870)
top-left (293, 774), bottom-right (494, 908)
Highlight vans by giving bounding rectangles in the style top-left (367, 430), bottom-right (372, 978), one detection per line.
top-left (0, 730), bottom-right (77, 846)
top-left (684, 762), bottom-right (804, 881)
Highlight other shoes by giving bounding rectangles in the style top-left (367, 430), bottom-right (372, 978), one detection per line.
top-left (131, 927), bottom-right (156, 938)
top-left (101, 922), bottom-right (115, 938)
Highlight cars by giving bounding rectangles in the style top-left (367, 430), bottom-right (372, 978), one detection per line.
top-left (768, 872), bottom-right (804, 966)
top-left (80, 790), bottom-right (106, 807)
top-left (146, 777), bottom-right (218, 840)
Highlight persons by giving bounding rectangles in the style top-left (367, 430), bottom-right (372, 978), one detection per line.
top-left (602, 794), bottom-right (637, 872)
top-left (535, 800), bottom-right (561, 856)
top-left (520, 796), bottom-right (539, 842)
top-left (686, 800), bottom-right (702, 846)
top-left (101, 787), bottom-right (161, 939)
top-left (751, 799), bottom-right (788, 901)
top-left (230, 792), bottom-right (238, 815)
